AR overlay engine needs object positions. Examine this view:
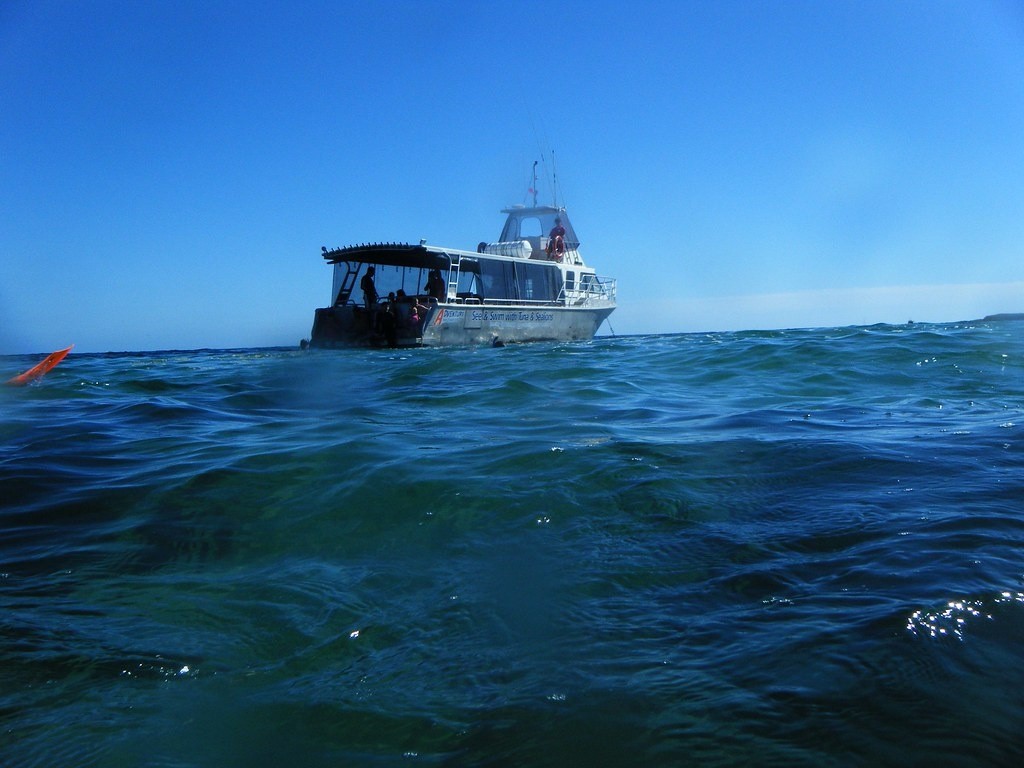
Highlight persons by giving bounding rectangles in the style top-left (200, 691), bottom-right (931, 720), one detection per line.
top-left (547, 218), bottom-right (565, 261)
top-left (361, 266), bottom-right (446, 341)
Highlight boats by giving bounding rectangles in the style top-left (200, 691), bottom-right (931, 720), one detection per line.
top-left (292, 153), bottom-right (621, 345)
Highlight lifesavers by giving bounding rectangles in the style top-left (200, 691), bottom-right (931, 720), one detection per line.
top-left (552, 235), bottom-right (564, 259)
